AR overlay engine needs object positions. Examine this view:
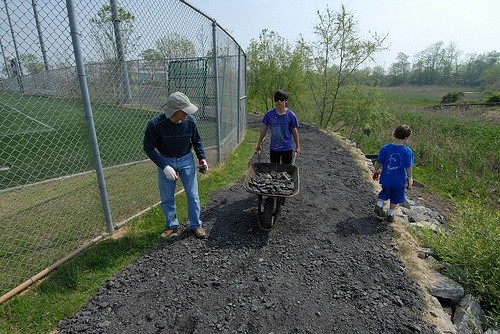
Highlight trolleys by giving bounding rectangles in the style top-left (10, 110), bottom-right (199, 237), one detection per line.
top-left (243, 146), bottom-right (301, 232)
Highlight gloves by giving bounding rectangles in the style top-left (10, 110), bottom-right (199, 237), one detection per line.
top-left (197, 159), bottom-right (209, 174)
top-left (372, 169), bottom-right (380, 181)
top-left (163, 165), bottom-right (179, 181)
top-left (406, 176), bottom-right (412, 190)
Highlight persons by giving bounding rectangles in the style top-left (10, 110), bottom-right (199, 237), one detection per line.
top-left (256, 91), bottom-right (301, 165)
top-left (372, 125), bottom-right (414, 223)
top-left (143, 92), bottom-right (209, 239)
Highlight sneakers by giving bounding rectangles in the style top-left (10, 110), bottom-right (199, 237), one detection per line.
top-left (161, 227), bottom-right (179, 239)
top-left (374, 205), bottom-right (385, 217)
top-left (194, 225), bottom-right (206, 238)
top-left (385, 210), bottom-right (395, 222)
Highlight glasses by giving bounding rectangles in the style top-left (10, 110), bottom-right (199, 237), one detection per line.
top-left (275, 98), bottom-right (287, 102)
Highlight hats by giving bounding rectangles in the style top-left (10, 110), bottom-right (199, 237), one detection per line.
top-left (164, 91), bottom-right (198, 118)
